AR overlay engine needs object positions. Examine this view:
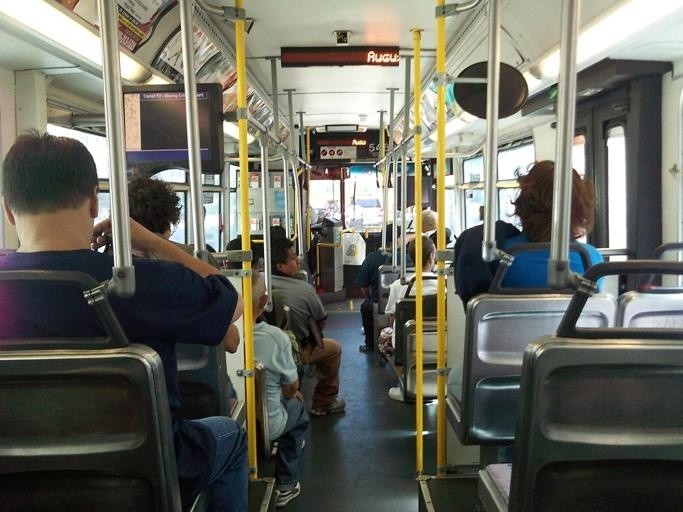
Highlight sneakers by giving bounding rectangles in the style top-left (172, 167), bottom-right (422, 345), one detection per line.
top-left (310, 399), bottom-right (346, 415)
top-left (388, 386), bottom-right (404, 402)
top-left (359, 345), bottom-right (373, 353)
top-left (273, 482), bottom-right (301, 507)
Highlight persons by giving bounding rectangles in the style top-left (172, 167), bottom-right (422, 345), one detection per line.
top-left (355, 209), bottom-right (453, 350)
top-left (307, 202), bottom-right (327, 245)
top-left (107, 176), bottom-right (344, 506)
top-left (465, 159), bottom-right (605, 294)
top-left (0, 127), bottom-right (249, 512)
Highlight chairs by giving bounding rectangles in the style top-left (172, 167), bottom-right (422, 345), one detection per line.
top-left (475, 261), bottom-right (683, 512)
top-left (373, 264), bottom-right (447, 404)
top-left (0, 264), bottom-right (306, 512)
top-left (445, 241), bottom-right (683, 447)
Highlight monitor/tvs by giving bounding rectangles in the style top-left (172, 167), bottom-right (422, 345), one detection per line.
top-left (122, 83), bottom-right (224, 174)
top-left (397, 177), bottom-right (433, 209)
